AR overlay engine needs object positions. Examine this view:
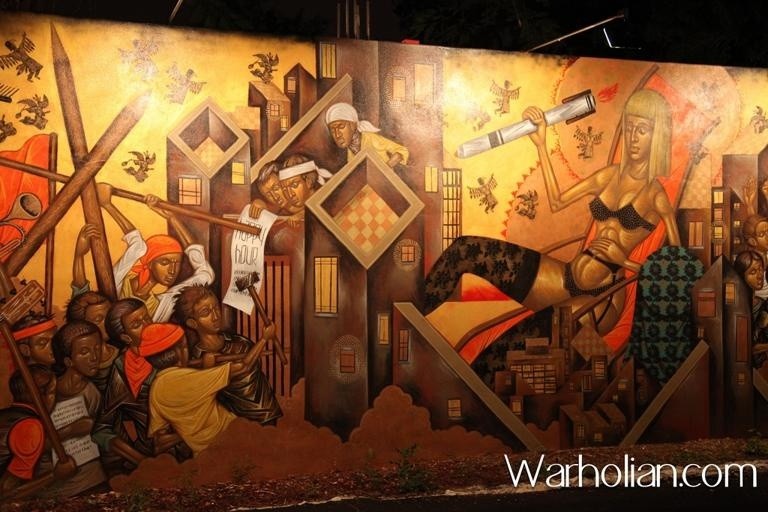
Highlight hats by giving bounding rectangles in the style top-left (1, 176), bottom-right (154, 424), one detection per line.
top-left (138, 320), bottom-right (187, 358)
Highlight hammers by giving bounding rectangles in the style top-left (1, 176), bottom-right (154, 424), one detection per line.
top-left (235, 272), bottom-right (288, 365)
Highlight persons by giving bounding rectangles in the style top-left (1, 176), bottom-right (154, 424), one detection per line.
top-left (734, 177), bottom-right (768, 366)
top-left (325, 103), bottom-right (410, 168)
top-left (420, 90), bottom-right (680, 386)
